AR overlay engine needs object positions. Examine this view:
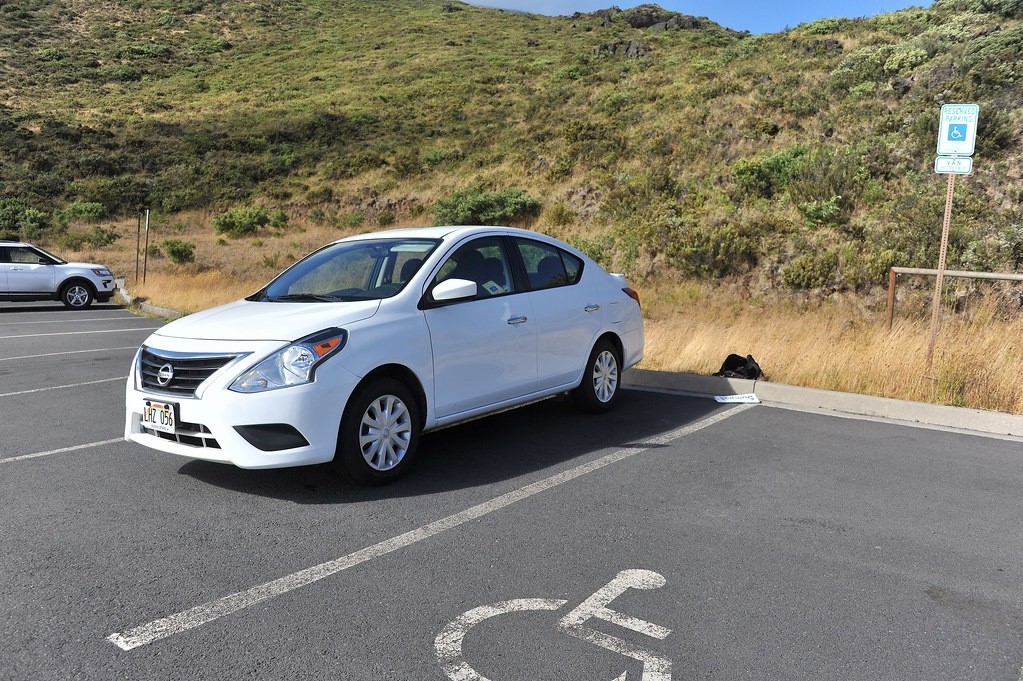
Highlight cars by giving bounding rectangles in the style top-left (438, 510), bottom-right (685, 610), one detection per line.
top-left (124, 224), bottom-right (645, 481)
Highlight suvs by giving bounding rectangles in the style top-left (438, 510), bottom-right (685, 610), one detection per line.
top-left (0, 242), bottom-right (117, 310)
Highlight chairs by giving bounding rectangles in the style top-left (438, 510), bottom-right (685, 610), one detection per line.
top-left (537, 256), bottom-right (565, 280)
top-left (399, 259), bottom-right (435, 284)
top-left (485, 257), bottom-right (504, 282)
top-left (445, 250), bottom-right (487, 281)
